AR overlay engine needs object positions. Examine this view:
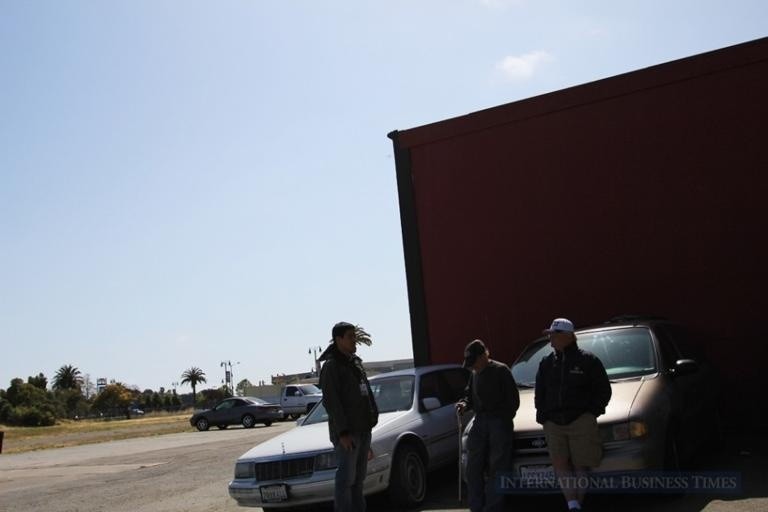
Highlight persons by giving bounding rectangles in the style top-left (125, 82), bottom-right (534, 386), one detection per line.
top-left (456, 338), bottom-right (520, 511)
top-left (534, 318), bottom-right (611, 512)
top-left (318, 321), bottom-right (380, 510)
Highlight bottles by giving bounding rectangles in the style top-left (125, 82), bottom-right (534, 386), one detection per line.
top-left (543, 318), bottom-right (575, 335)
top-left (462, 339), bottom-right (485, 368)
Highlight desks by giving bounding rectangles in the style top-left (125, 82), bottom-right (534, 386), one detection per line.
top-left (308, 345), bottom-right (322, 376)
top-left (220, 361), bottom-right (240, 396)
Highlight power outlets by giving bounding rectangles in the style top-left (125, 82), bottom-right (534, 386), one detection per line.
top-left (243, 383), bottom-right (323, 421)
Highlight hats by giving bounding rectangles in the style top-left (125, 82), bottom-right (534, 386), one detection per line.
top-left (461, 322), bottom-right (696, 496)
top-left (227, 362), bottom-right (473, 508)
top-left (189, 397), bottom-right (283, 432)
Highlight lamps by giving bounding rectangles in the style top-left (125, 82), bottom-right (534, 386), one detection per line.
top-left (375, 379), bottom-right (412, 413)
